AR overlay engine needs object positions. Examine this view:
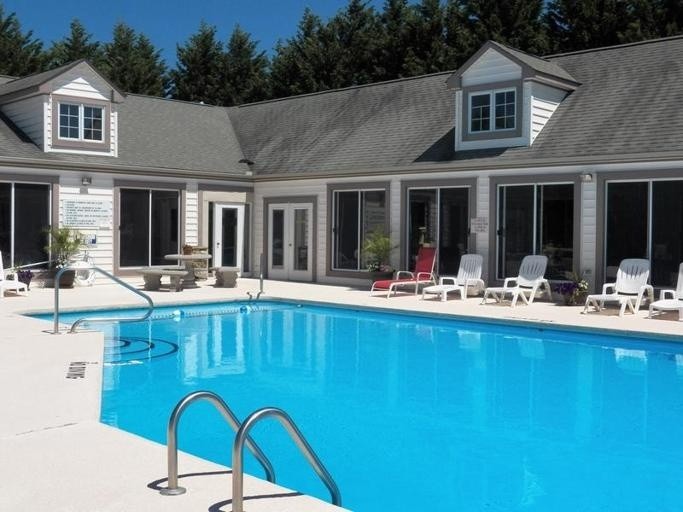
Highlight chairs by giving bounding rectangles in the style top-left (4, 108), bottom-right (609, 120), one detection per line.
top-left (648, 263), bottom-right (683, 321)
top-left (479, 255), bottom-right (552, 308)
top-left (369, 246), bottom-right (436, 299)
top-left (580, 258), bottom-right (654, 317)
top-left (422, 254), bottom-right (485, 302)
top-left (0, 250), bottom-right (28, 299)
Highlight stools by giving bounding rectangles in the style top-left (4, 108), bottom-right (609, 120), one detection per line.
top-left (137, 266), bottom-right (240, 292)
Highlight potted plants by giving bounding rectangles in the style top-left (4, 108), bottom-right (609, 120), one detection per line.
top-left (41, 223), bottom-right (84, 287)
top-left (6, 259), bottom-right (34, 291)
top-left (362, 224), bottom-right (396, 283)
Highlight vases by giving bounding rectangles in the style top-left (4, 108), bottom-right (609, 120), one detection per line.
top-left (575, 290), bottom-right (588, 306)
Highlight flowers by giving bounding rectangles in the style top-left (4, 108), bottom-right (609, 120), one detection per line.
top-left (553, 271), bottom-right (588, 305)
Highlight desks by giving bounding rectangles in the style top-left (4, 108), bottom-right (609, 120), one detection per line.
top-left (164, 254), bottom-right (212, 289)
